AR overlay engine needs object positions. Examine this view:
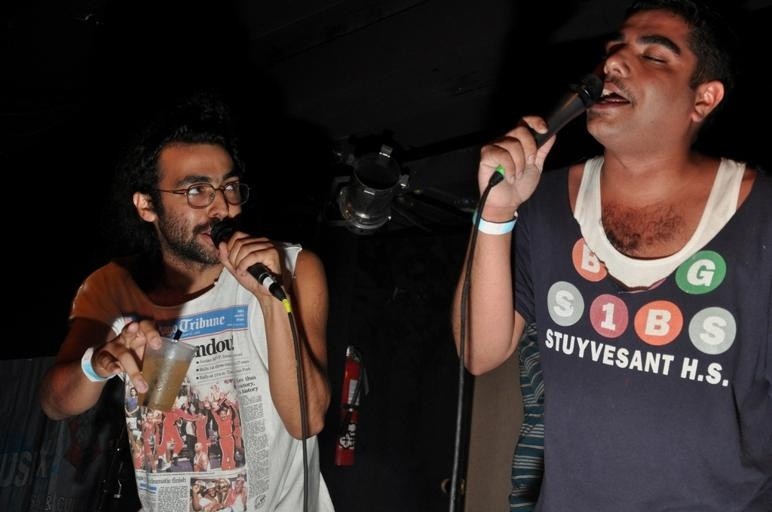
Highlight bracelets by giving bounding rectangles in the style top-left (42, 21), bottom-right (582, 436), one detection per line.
top-left (81, 345), bottom-right (118, 382)
top-left (472, 208), bottom-right (518, 236)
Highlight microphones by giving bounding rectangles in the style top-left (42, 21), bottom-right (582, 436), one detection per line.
top-left (211, 216), bottom-right (293, 313)
top-left (489, 73), bottom-right (603, 188)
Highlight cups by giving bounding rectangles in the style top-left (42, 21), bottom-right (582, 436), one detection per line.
top-left (136, 334), bottom-right (197, 413)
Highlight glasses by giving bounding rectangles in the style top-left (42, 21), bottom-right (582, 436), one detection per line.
top-left (154, 184), bottom-right (249, 209)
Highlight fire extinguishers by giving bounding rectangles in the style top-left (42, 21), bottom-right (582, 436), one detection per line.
top-left (335, 346), bottom-right (364, 466)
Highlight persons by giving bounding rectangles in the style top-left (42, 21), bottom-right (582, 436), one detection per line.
top-left (450, 2), bottom-right (772, 512)
top-left (38, 123), bottom-right (335, 511)
